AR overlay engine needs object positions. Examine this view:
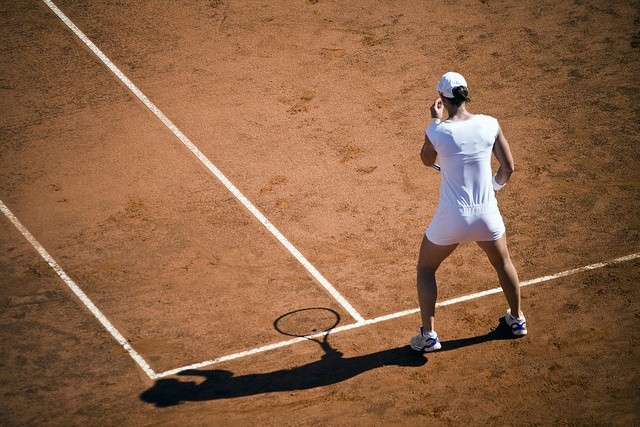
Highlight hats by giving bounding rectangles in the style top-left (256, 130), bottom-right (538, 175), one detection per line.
top-left (437, 72), bottom-right (467, 98)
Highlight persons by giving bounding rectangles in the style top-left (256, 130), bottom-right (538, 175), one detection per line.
top-left (410, 71), bottom-right (527, 352)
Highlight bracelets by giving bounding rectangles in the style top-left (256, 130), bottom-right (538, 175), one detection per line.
top-left (430, 118), bottom-right (440, 126)
top-left (492, 174), bottom-right (507, 191)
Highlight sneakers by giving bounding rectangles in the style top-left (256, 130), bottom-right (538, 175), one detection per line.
top-left (411, 327), bottom-right (441, 351)
top-left (504, 309), bottom-right (527, 336)
